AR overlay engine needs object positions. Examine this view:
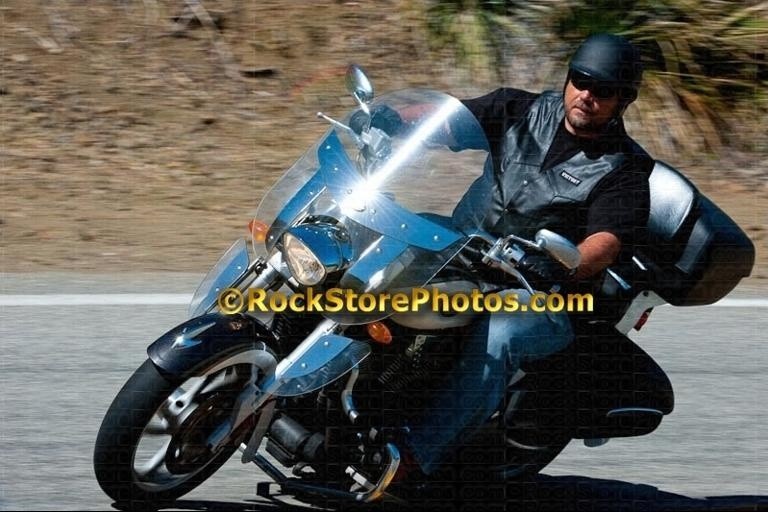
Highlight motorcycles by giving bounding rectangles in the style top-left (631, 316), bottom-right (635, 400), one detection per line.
top-left (94, 63), bottom-right (755, 508)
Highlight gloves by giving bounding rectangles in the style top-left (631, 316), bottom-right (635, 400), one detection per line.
top-left (349, 105), bottom-right (403, 138)
top-left (515, 247), bottom-right (568, 290)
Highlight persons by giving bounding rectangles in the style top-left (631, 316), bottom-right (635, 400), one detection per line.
top-left (350, 35), bottom-right (651, 485)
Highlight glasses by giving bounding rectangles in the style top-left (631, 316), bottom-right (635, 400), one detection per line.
top-left (569, 67), bottom-right (618, 101)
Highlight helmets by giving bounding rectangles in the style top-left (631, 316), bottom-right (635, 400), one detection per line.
top-left (571, 33), bottom-right (642, 89)
